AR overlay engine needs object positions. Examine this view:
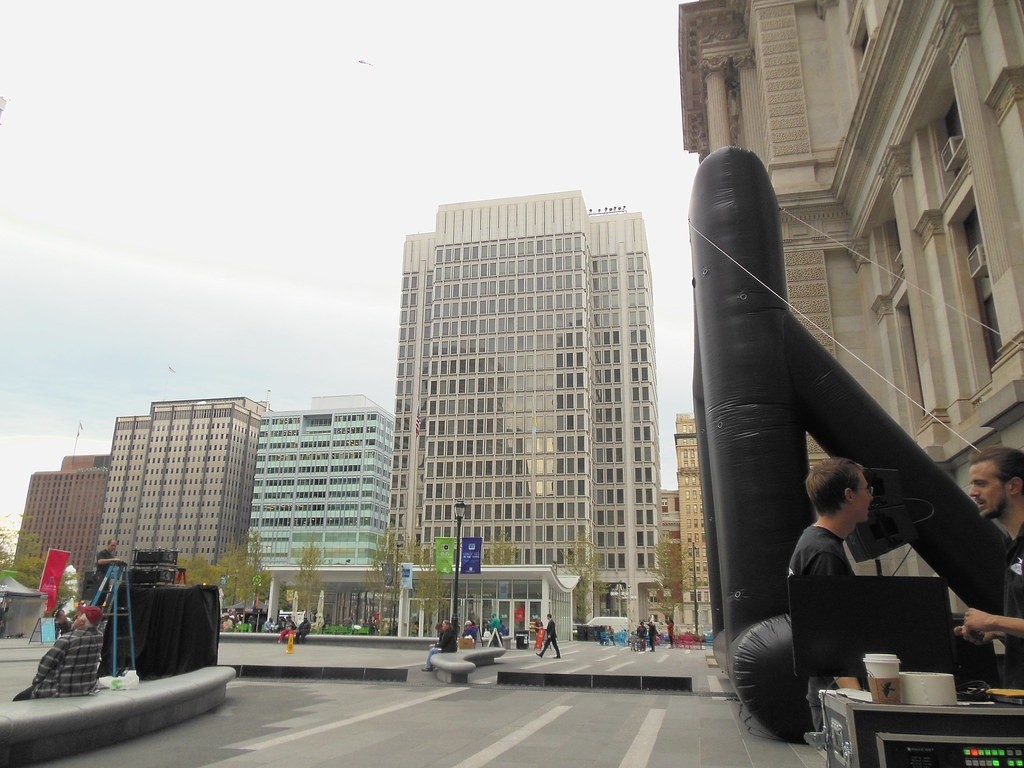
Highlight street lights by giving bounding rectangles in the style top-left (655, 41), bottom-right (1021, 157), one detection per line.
top-left (451, 500), bottom-right (464, 638)
top-left (688, 547), bottom-right (700, 635)
top-left (610, 584), bottom-right (628, 617)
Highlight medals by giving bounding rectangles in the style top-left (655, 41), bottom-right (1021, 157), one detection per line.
top-left (222, 598), bottom-right (269, 631)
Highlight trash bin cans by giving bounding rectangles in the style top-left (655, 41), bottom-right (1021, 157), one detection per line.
top-left (515, 630), bottom-right (530, 650)
top-left (576, 625), bottom-right (607, 642)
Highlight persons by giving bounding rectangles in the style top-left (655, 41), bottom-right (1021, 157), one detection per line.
top-left (221, 608), bottom-right (313, 644)
top-left (420, 612), bottom-right (507, 671)
top-left (786, 455), bottom-right (873, 732)
top-left (583, 621), bottom-right (660, 652)
top-left (54, 609), bottom-right (69, 639)
top-left (665, 616), bottom-right (677, 649)
top-left (10, 606), bottom-right (104, 701)
top-left (94, 539), bottom-right (117, 605)
top-left (322, 613), bottom-right (419, 637)
top-left (533, 614), bottom-right (564, 659)
top-left (954, 448), bottom-right (1024, 685)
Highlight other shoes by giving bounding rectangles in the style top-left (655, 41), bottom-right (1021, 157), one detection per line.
top-left (421, 668), bottom-right (433, 671)
top-left (536, 653), bottom-right (542, 658)
top-left (553, 656), bottom-right (560, 659)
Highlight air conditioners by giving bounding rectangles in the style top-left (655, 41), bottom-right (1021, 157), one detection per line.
top-left (968, 244), bottom-right (986, 279)
top-left (941, 136), bottom-right (964, 171)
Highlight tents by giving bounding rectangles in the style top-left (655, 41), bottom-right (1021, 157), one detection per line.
top-left (0, 576), bottom-right (41, 638)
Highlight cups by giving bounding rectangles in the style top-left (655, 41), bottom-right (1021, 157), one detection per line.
top-left (862, 654), bottom-right (902, 706)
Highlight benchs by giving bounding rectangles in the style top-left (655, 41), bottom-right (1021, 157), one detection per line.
top-left (0, 665), bottom-right (236, 768)
top-left (430, 646), bottom-right (505, 685)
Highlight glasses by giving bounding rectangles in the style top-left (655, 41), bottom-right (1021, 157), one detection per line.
top-left (842, 487), bottom-right (873, 498)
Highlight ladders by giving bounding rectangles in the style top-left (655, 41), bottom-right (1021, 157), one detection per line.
top-left (90, 561), bottom-right (135, 678)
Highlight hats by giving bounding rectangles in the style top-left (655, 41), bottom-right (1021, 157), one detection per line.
top-left (465, 621), bottom-right (472, 626)
top-left (78, 605), bottom-right (104, 625)
